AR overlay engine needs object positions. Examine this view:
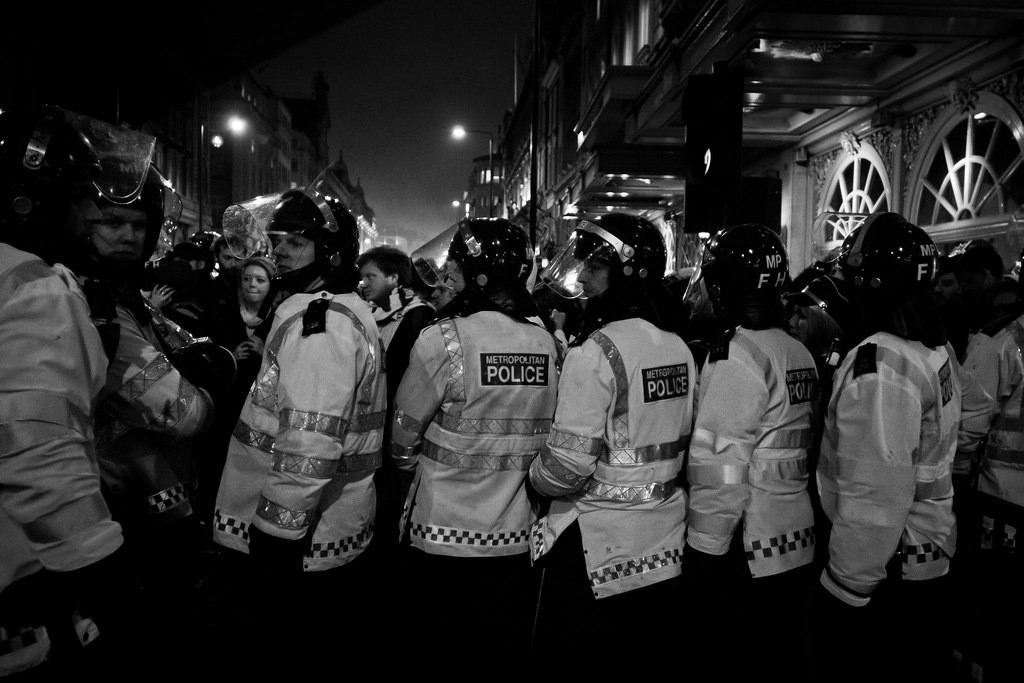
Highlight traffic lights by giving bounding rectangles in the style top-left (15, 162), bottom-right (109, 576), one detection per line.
top-left (688, 74), bottom-right (744, 188)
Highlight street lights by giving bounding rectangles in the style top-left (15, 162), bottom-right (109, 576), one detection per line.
top-left (450, 125), bottom-right (493, 217)
top-left (197, 111), bottom-right (253, 232)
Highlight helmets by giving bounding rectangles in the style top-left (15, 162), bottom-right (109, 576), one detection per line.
top-left (704, 224), bottom-right (786, 320)
top-left (573, 213), bottom-right (667, 300)
top-left (82, 160), bottom-right (165, 262)
top-left (449, 215), bottom-right (533, 301)
top-left (835, 214), bottom-right (939, 326)
top-left (0, 114), bottom-right (104, 234)
top-left (266, 189), bottom-right (361, 294)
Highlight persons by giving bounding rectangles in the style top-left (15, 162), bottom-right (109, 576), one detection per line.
top-left (1, 99), bottom-right (1024, 682)
